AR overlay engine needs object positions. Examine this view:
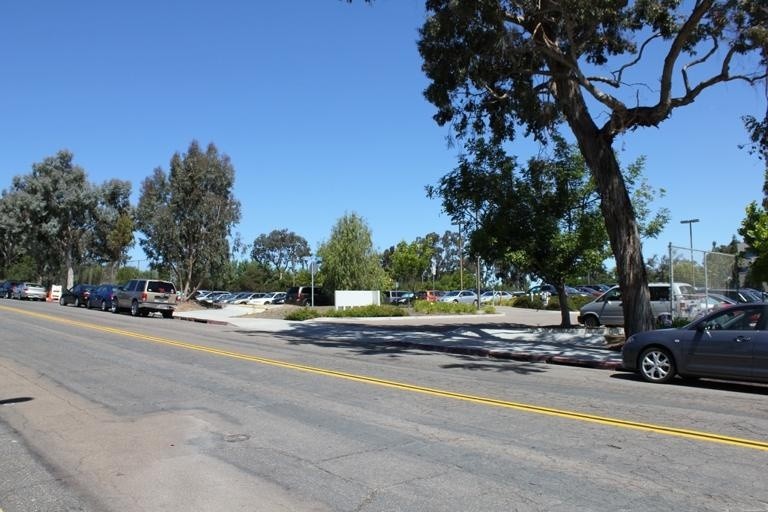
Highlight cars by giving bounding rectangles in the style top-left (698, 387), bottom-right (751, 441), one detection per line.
top-left (192, 285), bottom-right (333, 306)
top-left (381, 281), bottom-right (620, 307)
top-left (577, 277), bottom-right (767, 388)
top-left (0, 278), bottom-right (180, 319)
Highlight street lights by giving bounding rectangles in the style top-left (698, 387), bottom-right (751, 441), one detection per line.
top-left (451, 219), bottom-right (470, 290)
top-left (302, 254), bottom-right (321, 307)
top-left (679, 218), bottom-right (698, 290)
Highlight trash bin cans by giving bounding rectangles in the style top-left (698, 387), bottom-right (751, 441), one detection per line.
top-left (658, 312), bottom-right (672, 326)
